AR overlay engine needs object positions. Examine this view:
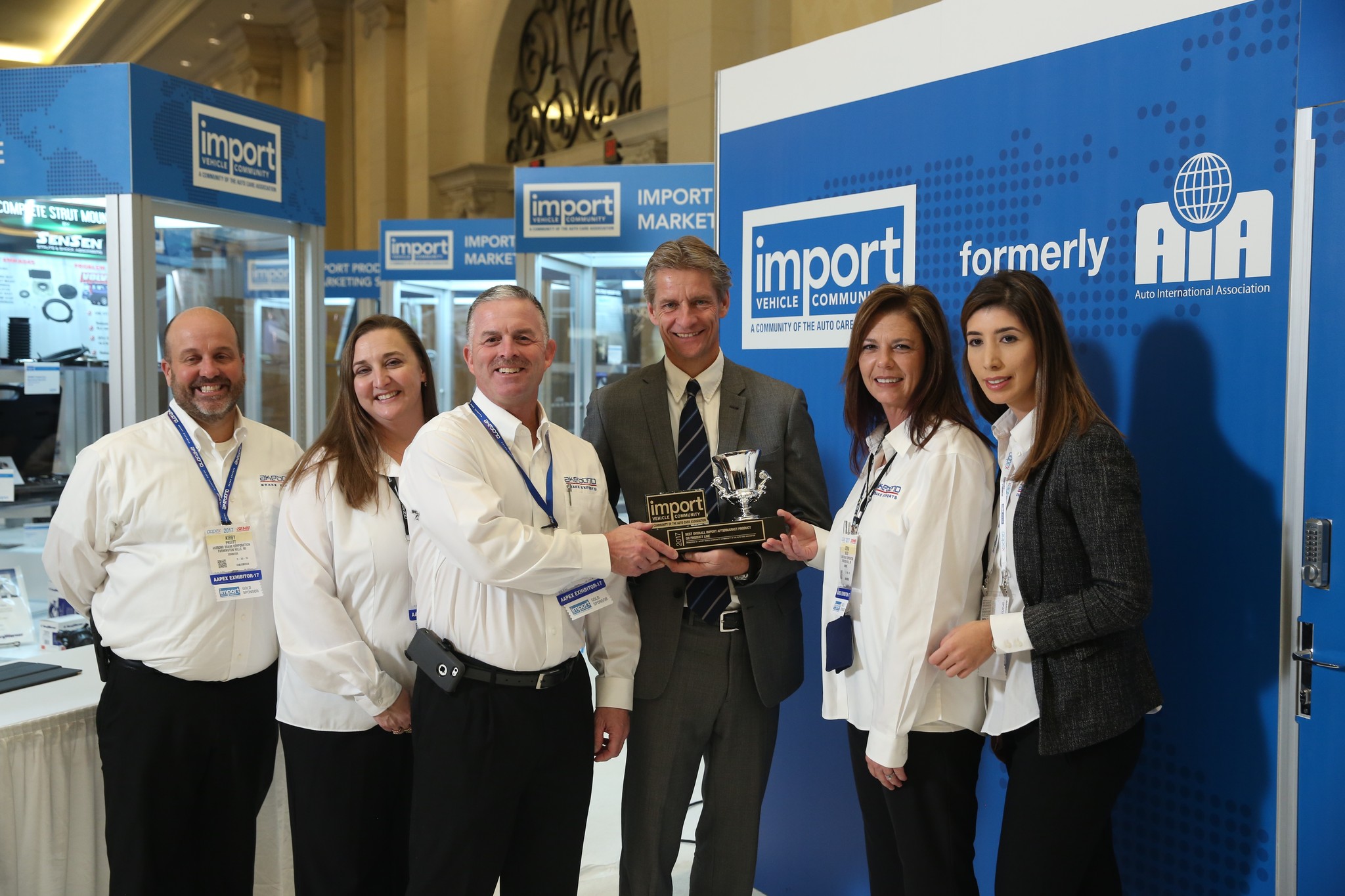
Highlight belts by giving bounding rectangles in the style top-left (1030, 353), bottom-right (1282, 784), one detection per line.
top-left (462, 653), bottom-right (581, 689)
top-left (681, 607), bottom-right (744, 633)
top-left (109, 655), bottom-right (159, 672)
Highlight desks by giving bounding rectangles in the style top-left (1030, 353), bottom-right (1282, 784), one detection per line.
top-left (0, 640), bottom-right (293, 895)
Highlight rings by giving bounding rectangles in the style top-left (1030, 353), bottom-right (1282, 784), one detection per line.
top-left (390, 726), bottom-right (411, 736)
top-left (884, 772), bottom-right (895, 781)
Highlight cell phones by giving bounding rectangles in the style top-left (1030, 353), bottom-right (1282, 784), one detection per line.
top-left (407, 630), bottom-right (465, 693)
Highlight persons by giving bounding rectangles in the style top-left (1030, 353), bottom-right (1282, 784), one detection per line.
top-left (579, 236), bottom-right (831, 896)
top-left (271, 315), bottom-right (441, 896)
top-left (400, 285), bottom-right (679, 896)
top-left (761, 269), bottom-right (1158, 896)
top-left (44, 307), bottom-right (308, 896)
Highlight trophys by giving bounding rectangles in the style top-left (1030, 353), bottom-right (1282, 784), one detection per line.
top-left (653, 450), bottom-right (786, 553)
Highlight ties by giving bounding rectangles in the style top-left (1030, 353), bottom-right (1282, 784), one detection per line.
top-left (677, 380), bottom-right (732, 625)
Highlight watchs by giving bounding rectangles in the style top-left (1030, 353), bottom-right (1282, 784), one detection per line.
top-left (991, 638), bottom-right (996, 652)
